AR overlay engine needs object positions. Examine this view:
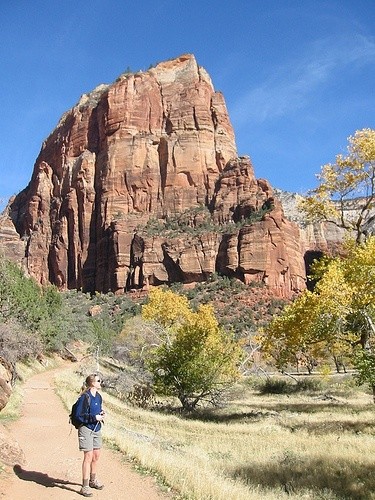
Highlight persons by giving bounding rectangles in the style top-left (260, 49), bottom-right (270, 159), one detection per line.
top-left (73, 374), bottom-right (105, 497)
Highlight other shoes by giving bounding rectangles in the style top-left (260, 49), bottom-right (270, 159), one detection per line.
top-left (80, 488), bottom-right (93, 497)
top-left (89, 480), bottom-right (104, 489)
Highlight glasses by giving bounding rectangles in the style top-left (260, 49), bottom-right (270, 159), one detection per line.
top-left (97, 380), bottom-right (100, 382)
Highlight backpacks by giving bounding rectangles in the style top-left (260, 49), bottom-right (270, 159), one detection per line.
top-left (68, 392), bottom-right (90, 429)
